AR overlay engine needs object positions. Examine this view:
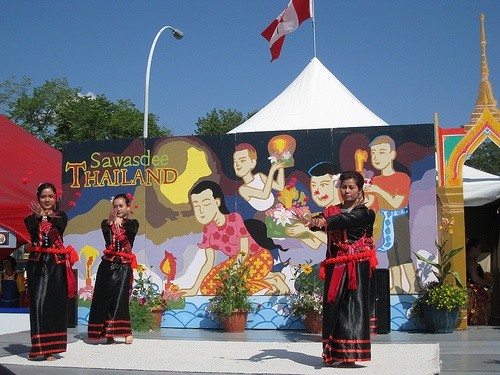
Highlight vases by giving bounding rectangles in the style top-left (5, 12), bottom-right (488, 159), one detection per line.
top-left (302, 309), bottom-right (322, 335)
top-left (220, 308), bottom-right (249, 333)
top-left (152, 309), bottom-right (162, 327)
top-left (420, 301), bottom-right (461, 334)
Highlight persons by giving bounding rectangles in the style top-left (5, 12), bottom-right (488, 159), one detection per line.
top-left (87, 194), bottom-right (140, 344)
top-left (23, 183), bottom-right (76, 361)
top-left (465, 238), bottom-right (494, 325)
top-left (296, 170), bottom-right (376, 368)
top-left (0, 256), bottom-right (26, 308)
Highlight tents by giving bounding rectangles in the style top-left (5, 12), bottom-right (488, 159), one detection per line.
top-left (226, 55), bottom-right (500, 213)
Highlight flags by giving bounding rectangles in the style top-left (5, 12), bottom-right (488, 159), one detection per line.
top-left (261, 0), bottom-right (314, 62)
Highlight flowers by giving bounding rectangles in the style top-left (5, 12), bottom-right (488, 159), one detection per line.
top-left (411, 217), bottom-right (475, 315)
top-left (130, 267), bottom-right (167, 333)
top-left (210, 251), bottom-right (261, 317)
top-left (284, 259), bottom-right (323, 320)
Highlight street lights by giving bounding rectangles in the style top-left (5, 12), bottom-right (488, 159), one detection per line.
top-left (142, 25), bottom-right (185, 140)
